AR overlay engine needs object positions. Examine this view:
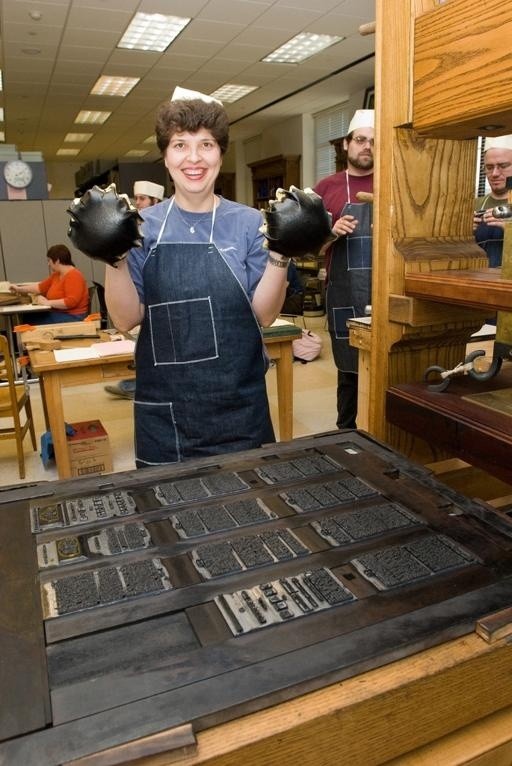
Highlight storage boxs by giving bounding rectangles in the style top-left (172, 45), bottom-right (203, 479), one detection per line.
top-left (63, 417), bottom-right (115, 478)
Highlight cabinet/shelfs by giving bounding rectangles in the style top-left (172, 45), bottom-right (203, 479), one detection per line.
top-left (74, 163), bottom-right (167, 199)
top-left (328, 135), bottom-right (348, 173)
top-left (364, 2), bottom-right (512, 485)
top-left (244, 151), bottom-right (301, 211)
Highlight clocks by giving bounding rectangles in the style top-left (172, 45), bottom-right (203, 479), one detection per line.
top-left (4, 159), bottom-right (34, 189)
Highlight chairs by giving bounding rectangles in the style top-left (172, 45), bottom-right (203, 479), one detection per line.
top-left (87, 287), bottom-right (99, 314)
top-left (0, 333), bottom-right (36, 479)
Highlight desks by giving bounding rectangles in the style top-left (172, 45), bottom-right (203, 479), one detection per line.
top-left (21, 313), bottom-right (303, 480)
top-left (1, 300), bottom-right (52, 388)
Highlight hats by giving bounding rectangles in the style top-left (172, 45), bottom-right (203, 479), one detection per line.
top-left (132, 180), bottom-right (166, 201)
top-left (348, 108), bottom-right (375, 133)
top-left (484, 134), bottom-right (512, 151)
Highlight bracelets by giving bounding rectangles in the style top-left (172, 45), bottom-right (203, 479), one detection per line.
top-left (267, 254), bottom-right (290, 268)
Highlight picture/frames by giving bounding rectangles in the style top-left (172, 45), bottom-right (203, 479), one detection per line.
top-left (362, 84), bottom-right (375, 110)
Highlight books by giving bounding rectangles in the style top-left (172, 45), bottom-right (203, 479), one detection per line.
top-left (53, 339), bottom-right (137, 364)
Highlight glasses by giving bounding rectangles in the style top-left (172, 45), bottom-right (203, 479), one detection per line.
top-left (352, 136), bottom-right (374, 147)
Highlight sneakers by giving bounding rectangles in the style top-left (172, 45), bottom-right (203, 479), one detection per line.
top-left (104, 383), bottom-right (136, 401)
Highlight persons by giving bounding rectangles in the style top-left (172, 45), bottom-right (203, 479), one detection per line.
top-left (9, 242), bottom-right (89, 323)
top-left (473, 139), bottom-right (512, 267)
top-left (313, 106), bottom-right (375, 428)
top-left (62, 97), bottom-right (340, 470)
top-left (129, 180), bottom-right (162, 211)
